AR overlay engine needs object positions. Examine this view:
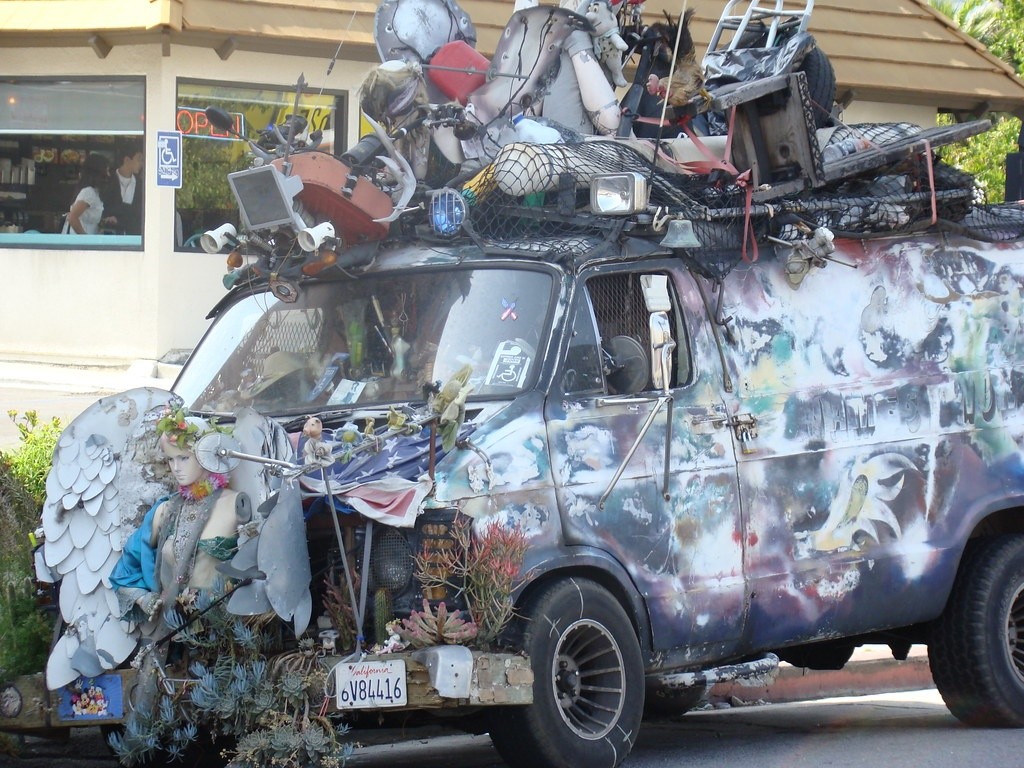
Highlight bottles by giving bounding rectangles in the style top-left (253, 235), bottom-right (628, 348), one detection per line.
top-left (823, 138), bottom-right (870, 162)
top-left (521, 190), bottom-right (545, 227)
top-left (513, 114), bottom-right (566, 144)
top-left (428, 40), bottom-right (492, 107)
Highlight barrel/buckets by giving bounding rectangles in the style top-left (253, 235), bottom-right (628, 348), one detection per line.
top-left (341, 133), bottom-right (387, 173)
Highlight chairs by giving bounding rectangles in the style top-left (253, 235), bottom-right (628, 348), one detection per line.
top-left (675, 70), bottom-right (996, 203)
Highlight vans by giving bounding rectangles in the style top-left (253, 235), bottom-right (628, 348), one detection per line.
top-left (0, 214), bottom-right (1023, 768)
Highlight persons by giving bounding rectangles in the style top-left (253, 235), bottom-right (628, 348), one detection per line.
top-left (61, 143), bottom-right (143, 234)
top-left (109, 417), bottom-right (252, 623)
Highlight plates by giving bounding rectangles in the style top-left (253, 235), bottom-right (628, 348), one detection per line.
top-left (60, 150), bottom-right (80, 165)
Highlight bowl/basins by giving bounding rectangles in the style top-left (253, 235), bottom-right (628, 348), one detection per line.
top-left (42, 151), bottom-right (54, 162)
top-left (34, 155), bottom-right (43, 163)
